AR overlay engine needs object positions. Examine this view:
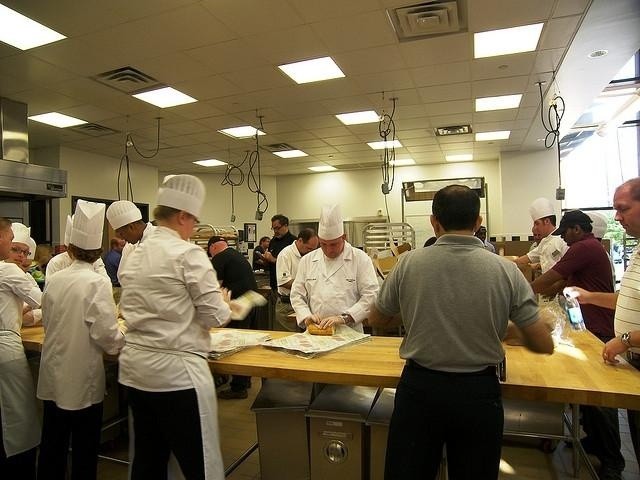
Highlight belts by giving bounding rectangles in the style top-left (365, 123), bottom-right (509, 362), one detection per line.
top-left (624, 350), bottom-right (639, 364)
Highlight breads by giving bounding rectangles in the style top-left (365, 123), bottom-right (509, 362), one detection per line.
top-left (308, 324), bottom-right (335, 335)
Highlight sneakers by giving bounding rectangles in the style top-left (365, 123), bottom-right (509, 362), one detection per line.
top-left (246, 383), bottom-right (251, 389)
top-left (216, 389), bottom-right (249, 399)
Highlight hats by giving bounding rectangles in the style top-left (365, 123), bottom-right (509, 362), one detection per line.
top-left (64, 214), bottom-right (74, 248)
top-left (154, 174), bottom-right (207, 218)
top-left (105, 200), bottom-right (143, 231)
top-left (549, 210), bottom-right (593, 236)
top-left (316, 204), bottom-right (345, 241)
top-left (206, 235), bottom-right (228, 257)
top-left (10, 221), bottom-right (31, 247)
top-left (528, 198), bottom-right (556, 221)
top-left (70, 198), bottom-right (107, 252)
top-left (585, 212), bottom-right (609, 238)
top-left (26, 237), bottom-right (37, 261)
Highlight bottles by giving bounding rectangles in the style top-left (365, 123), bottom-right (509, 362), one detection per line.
top-left (565, 293), bottom-right (586, 332)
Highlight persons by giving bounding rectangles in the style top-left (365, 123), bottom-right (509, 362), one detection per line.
top-left (1, 219), bottom-right (42, 479)
top-left (4, 222), bottom-right (32, 265)
top-left (474, 227), bottom-right (495, 254)
top-left (513, 196), bottom-right (568, 302)
top-left (253, 236), bottom-right (270, 271)
top-left (368, 184), bottom-right (555, 479)
top-left (585, 213), bottom-right (616, 293)
top-left (106, 200), bottom-right (158, 290)
top-left (119, 173), bottom-right (233, 478)
top-left (289, 203), bottom-right (379, 333)
top-left (37, 199), bottom-right (126, 480)
top-left (530, 225), bottom-right (543, 280)
top-left (207, 235), bottom-right (258, 400)
top-left (45, 213), bottom-right (113, 290)
top-left (532, 210), bottom-right (625, 480)
top-left (275, 229), bottom-right (319, 333)
top-left (561, 177), bottom-right (640, 474)
top-left (103, 238), bottom-right (124, 287)
top-left (264, 214), bottom-right (298, 305)
top-left (20, 237), bottom-right (43, 329)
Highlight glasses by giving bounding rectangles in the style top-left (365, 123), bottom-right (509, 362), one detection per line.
top-left (271, 224), bottom-right (283, 231)
top-left (11, 246), bottom-right (33, 256)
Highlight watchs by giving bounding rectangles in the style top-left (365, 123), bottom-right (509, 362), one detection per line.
top-left (343, 315), bottom-right (349, 324)
top-left (620, 331), bottom-right (632, 347)
